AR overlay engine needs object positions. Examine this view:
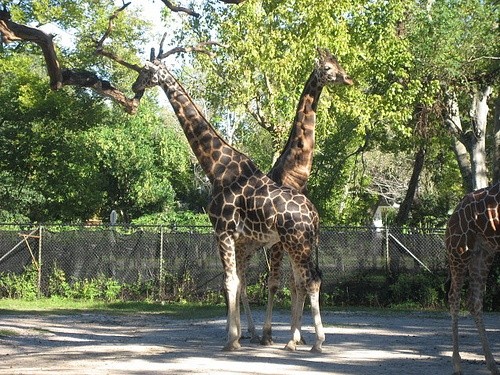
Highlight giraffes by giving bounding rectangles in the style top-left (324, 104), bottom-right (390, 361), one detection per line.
top-left (132, 58), bottom-right (325, 353)
top-left (224, 47), bottom-right (352, 345)
top-left (445, 178), bottom-right (500, 375)
